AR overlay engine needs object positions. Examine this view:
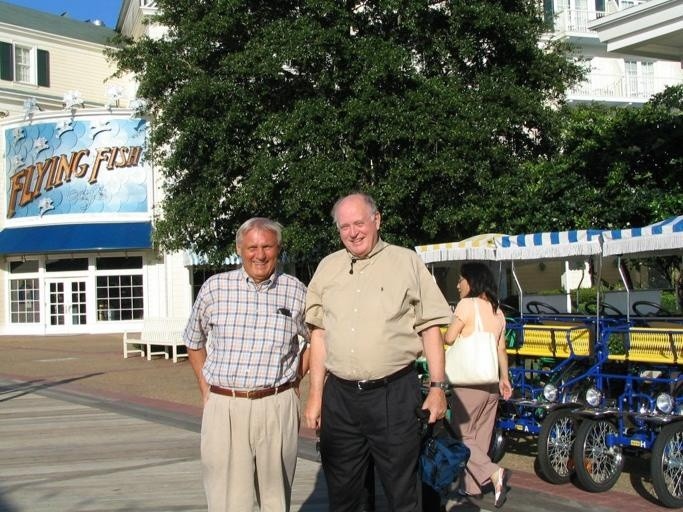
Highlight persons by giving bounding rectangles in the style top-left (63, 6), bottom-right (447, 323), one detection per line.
top-left (182, 217), bottom-right (307, 511)
top-left (441, 260), bottom-right (511, 509)
top-left (303, 190), bottom-right (450, 511)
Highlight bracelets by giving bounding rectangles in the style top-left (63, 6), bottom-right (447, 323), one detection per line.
top-left (431, 380), bottom-right (448, 390)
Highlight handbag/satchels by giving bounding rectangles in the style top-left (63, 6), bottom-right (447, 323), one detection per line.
top-left (443, 331), bottom-right (501, 388)
top-left (415, 405), bottom-right (471, 490)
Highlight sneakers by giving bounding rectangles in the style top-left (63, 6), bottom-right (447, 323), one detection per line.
top-left (457, 487), bottom-right (483, 500)
top-left (492, 466), bottom-right (511, 508)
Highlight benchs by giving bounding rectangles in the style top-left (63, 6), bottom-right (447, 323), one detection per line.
top-left (123, 319), bottom-right (190, 363)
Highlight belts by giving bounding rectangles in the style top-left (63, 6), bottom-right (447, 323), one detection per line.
top-left (209, 382), bottom-right (293, 400)
top-left (326, 361), bottom-right (414, 391)
